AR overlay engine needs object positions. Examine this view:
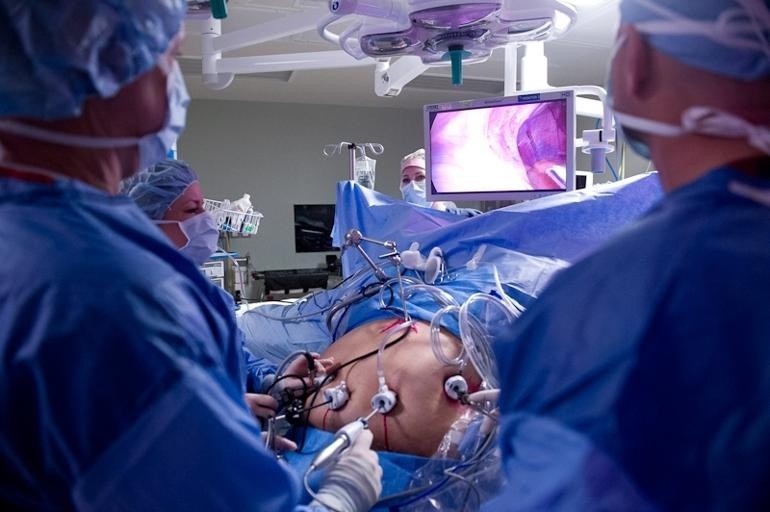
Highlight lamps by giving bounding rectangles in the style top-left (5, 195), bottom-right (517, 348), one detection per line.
top-left (319, 0), bottom-right (577, 85)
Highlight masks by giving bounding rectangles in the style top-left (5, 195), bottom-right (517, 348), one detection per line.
top-left (82, 50), bottom-right (190, 177)
top-left (153, 211), bottom-right (219, 269)
top-left (604, 38), bottom-right (681, 160)
top-left (398, 178), bottom-right (433, 210)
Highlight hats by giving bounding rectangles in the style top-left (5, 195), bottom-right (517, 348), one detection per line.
top-left (399, 148), bottom-right (426, 172)
top-left (620, 1), bottom-right (770, 82)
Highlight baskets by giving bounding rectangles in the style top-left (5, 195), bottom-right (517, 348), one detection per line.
top-left (205, 199), bottom-right (262, 235)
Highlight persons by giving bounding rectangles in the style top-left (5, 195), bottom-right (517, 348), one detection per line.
top-left (0, 1), bottom-right (384, 512)
top-left (119, 156), bottom-right (334, 420)
top-left (399, 148), bottom-right (484, 218)
top-left (467, 0), bottom-right (770, 512)
top-left (297, 306), bottom-right (488, 461)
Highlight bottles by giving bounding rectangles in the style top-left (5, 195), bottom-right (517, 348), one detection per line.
top-left (231, 193), bottom-right (252, 231)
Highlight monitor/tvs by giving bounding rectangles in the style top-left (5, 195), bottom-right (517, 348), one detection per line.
top-left (574, 169), bottom-right (593, 190)
top-left (423, 89), bottom-right (577, 202)
